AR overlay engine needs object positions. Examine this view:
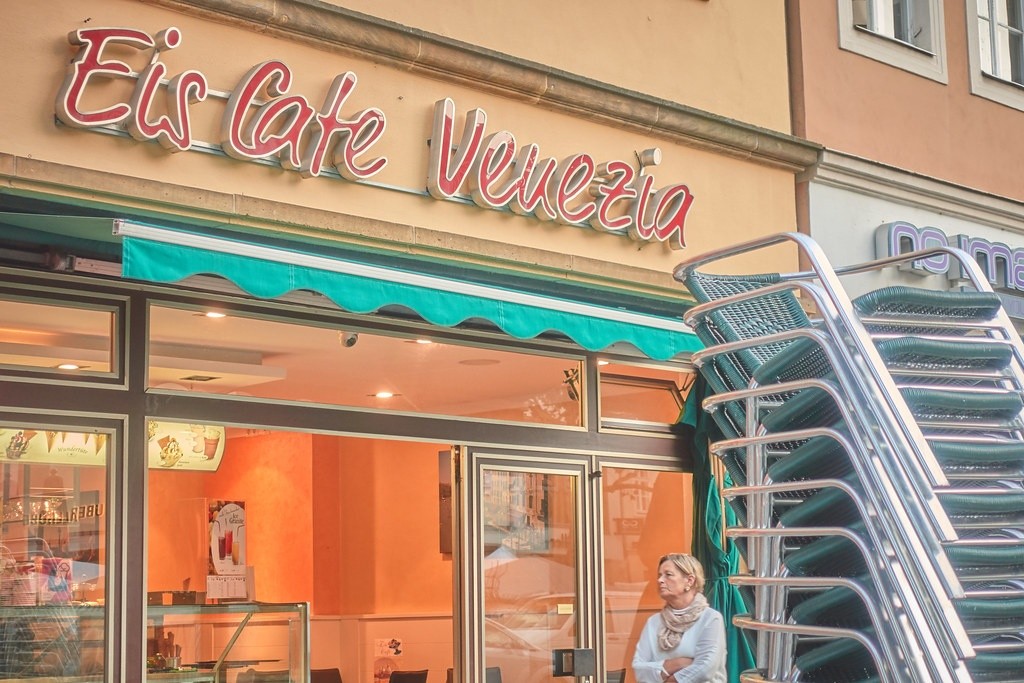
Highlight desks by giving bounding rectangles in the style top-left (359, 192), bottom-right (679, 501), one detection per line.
top-left (182, 659), bottom-right (281, 683)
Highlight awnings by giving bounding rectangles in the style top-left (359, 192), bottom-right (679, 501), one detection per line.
top-left (0, 210), bottom-right (710, 361)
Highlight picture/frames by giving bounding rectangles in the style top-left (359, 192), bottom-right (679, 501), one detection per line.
top-left (28, 490), bottom-right (99, 584)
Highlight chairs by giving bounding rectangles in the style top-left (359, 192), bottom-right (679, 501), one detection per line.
top-left (671, 231), bottom-right (1024, 683)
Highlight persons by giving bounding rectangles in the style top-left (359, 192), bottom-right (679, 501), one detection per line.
top-left (632, 552), bottom-right (728, 683)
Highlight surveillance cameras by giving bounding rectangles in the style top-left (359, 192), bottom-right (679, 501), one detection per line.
top-left (338, 330), bottom-right (358, 347)
